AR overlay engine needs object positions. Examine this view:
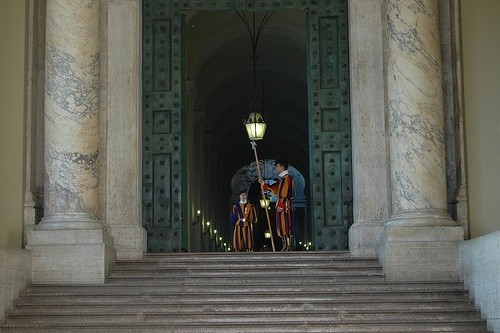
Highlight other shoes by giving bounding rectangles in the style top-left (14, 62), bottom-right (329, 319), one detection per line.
top-left (279, 240), bottom-right (286, 253)
top-left (284, 240), bottom-right (292, 251)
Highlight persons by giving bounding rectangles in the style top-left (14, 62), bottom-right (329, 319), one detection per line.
top-left (258, 159), bottom-right (295, 251)
top-left (231, 191), bottom-right (258, 253)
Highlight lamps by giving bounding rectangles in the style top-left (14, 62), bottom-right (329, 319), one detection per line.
top-left (232, 8), bottom-right (277, 142)
top-left (263, 229), bottom-right (271, 239)
top-left (256, 163), bottom-right (272, 208)
